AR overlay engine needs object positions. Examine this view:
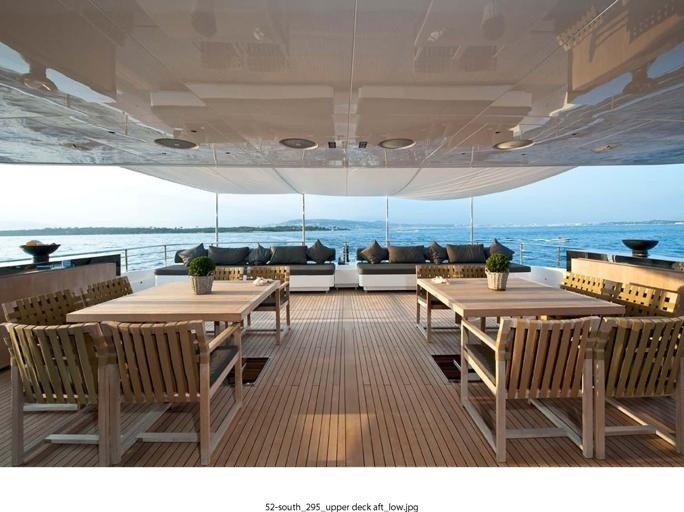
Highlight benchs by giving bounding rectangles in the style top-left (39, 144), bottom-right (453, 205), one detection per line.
top-left (153, 249), bottom-right (335, 294)
top-left (356, 248), bottom-right (532, 294)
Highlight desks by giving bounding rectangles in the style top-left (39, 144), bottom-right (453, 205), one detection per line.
top-left (417, 276), bottom-right (627, 387)
top-left (66, 278), bottom-right (281, 385)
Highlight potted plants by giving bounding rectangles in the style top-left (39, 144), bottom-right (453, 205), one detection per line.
top-left (483, 253), bottom-right (513, 290)
top-left (187, 256), bottom-right (216, 295)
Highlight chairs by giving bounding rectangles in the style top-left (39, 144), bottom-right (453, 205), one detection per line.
top-left (459, 314), bottom-right (684, 462)
top-left (0, 320), bottom-right (247, 466)
top-left (415, 264), bottom-right (514, 342)
top-left (1, 275), bottom-right (133, 397)
top-left (541, 270), bottom-right (680, 321)
top-left (214, 265), bottom-right (290, 345)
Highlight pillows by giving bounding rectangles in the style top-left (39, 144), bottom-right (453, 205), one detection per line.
top-left (178, 239), bottom-right (336, 268)
top-left (360, 239), bottom-right (515, 263)
